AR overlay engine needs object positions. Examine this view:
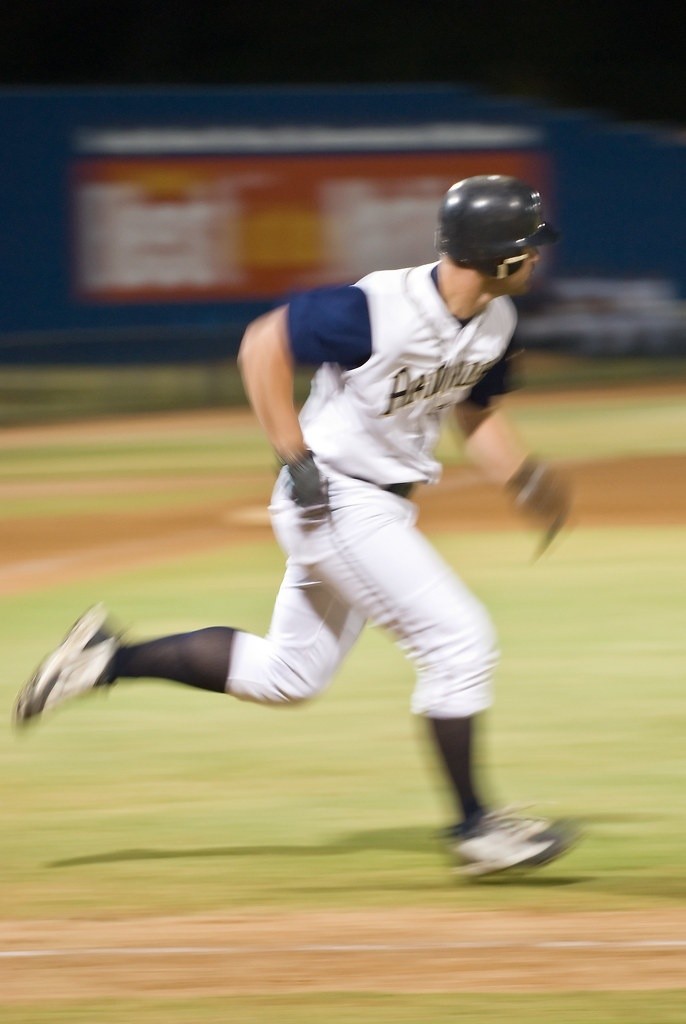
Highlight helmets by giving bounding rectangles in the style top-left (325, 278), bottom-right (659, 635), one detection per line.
top-left (434, 174), bottom-right (552, 280)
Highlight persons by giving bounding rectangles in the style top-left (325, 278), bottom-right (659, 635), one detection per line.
top-left (16, 171), bottom-right (585, 883)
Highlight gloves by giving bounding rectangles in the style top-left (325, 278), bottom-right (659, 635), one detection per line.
top-left (287, 452), bottom-right (326, 516)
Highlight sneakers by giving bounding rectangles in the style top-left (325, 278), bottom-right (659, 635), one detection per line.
top-left (445, 809), bottom-right (585, 883)
top-left (16, 602), bottom-right (122, 719)
